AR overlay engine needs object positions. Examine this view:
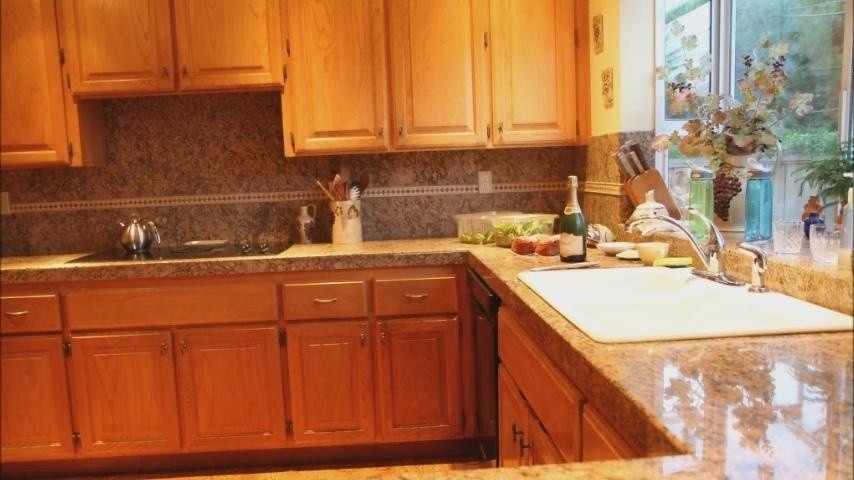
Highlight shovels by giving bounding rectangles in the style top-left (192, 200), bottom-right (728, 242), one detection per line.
top-left (333, 174), bottom-right (347, 201)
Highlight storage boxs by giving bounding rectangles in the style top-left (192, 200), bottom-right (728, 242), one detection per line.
top-left (454, 208), bottom-right (560, 248)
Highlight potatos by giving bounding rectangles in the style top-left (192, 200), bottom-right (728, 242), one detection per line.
top-left (511, 234), bottom-right (561, 257)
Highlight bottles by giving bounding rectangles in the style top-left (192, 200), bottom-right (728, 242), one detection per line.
top-left (295, 203), bottom-right (318, 244)
top-left (687, 166), bottom-right (712, 241)
top-left (560, 175), bottom-right (587, 262)
top-left (742, 165), bottom-right (778, 241)
top-left (803, 194), bottom-right (827, 238)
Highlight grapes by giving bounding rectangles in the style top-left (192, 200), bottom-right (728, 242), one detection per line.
top-left (710, 137), bottom-right (727, 171)
top-left (712, 173), bottom-right (742, 222)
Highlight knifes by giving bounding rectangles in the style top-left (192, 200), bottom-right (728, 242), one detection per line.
top-left (612, 140), bottom-right (649, 177)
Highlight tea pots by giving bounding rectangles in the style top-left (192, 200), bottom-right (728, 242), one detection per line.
top-left (116, 213), bottom-right (160, 254)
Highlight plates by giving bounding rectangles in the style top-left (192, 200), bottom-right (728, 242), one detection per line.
top-left (595, 241), bottom-right (635, 255)
top-left (185, 241), bottom-right (227, 250)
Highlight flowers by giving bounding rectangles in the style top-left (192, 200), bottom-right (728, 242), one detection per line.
top-left (646, 37), bottom-right (814, 177)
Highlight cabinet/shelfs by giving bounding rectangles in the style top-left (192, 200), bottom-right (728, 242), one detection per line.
top-left (282, 271), bottom-right (468, 450)
top-left (496, 306), bottom-right (644, 469)
top-left (61, 274), bottom-right (289, 461)
top-left (490, 1), bottom-right (578, 149)
top-left (0, 283), bottom-right (77, 464)
top-left (0, 1), bottom-right (109, 168)
top-left (279, 0), bottom-right (491, 158)
top-left (61, 1), bottom-right (284, 100)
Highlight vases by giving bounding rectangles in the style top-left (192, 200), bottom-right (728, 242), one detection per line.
top-left (714, 154), bottom-right (748, 232)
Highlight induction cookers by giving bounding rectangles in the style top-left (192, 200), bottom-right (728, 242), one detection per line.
top-left (63, 242), bottom-right (293, 265)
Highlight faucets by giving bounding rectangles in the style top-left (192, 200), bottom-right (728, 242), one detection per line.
top-left (624, 206), bottom-right (728, 282)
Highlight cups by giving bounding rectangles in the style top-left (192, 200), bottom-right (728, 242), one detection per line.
top-left (772, 221), bottom-right (808, 254)
top-left (810, 224), bottom-right (837, 264)
top-left (637, 242), bottom-right (670, 266)
top-left (326, 199), bottom-right (363, 244)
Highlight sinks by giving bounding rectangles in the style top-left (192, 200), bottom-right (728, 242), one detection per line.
top-left (516, 266), bottom-right (854, 345)
